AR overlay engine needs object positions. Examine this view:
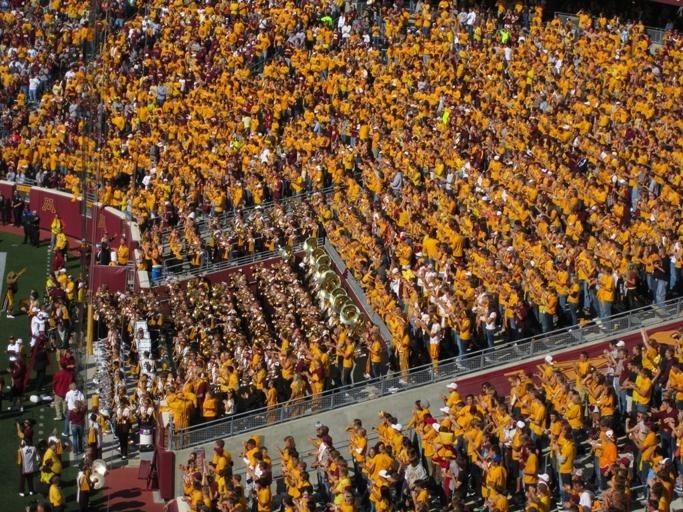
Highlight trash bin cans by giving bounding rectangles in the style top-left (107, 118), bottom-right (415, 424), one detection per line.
top-left (140, 423), bottom-right (154, 452)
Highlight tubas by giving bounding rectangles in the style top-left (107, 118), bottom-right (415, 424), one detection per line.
top-left (303, 238), bottom-right (361, 337)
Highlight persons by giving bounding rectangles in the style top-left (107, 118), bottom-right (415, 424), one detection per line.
top-left (0, 337), bottom-right (331, 512)
top-left (306, 411), bottom-right (434, 511)
top-left (533, 329), bottom-right (683, 512)
top-left (407, 385), bottom-right (552, 511)
top-left (1, 0), bottom-right (682, 385)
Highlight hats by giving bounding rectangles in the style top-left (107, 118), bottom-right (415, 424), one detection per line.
top-left (617, 456), bottom-right (630, 468)
top-left (446, 382), bottom-right (457, 390)
top-left (391, 423), bottom-right (402, 431)
top-left (545, 355), bottom-right (553, 364)
top-left (537, 473), bottom-right (550, 481)
top-left (423, 417), bottom-right (436, 424)
top-left (432, 422), bottom-right (440, 432)
top-left (440, 407), bottom-right (449, 415)
top-left (378, 469), bottom-right (391, 479)
top-left (605, 429), bottom-right (615, 442)
top-left (615, 339), bottom-right (625, 347)
top-left (490, 454), bottom-right (501, 462)
top-left (516, 420), bottom-right (526, 429)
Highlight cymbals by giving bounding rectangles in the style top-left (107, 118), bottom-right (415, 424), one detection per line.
top-left (92, 459), bottom-right (106, 475)
top-left (90, 472), bottom-right (104, 489)
